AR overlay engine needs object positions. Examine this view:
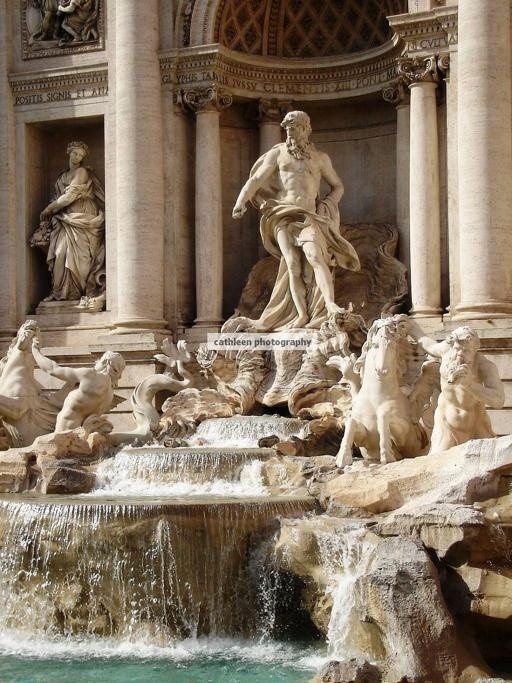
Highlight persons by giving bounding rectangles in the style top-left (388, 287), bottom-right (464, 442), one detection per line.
top-left (232, 109), bottom-right (344, 328)
top-left (38, 140), bottom-right (100, 303)
top-left (32, 338), bottom-right (127, 433)
top-left (392, 313), bottom-right (506, 456)
